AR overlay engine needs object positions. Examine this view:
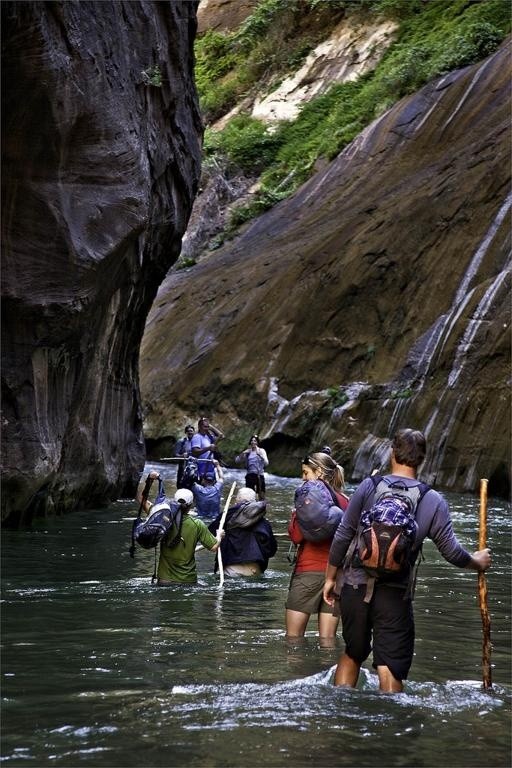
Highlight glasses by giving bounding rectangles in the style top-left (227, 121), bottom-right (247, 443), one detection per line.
top-left (301, 458), bottom-right (318, 467)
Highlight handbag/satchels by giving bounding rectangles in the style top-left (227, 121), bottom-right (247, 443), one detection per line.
top-left (227, 501), bottom-right (266, 530)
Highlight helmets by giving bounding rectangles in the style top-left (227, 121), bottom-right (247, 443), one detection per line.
top-left (322, 446), bottom-right (331, 453)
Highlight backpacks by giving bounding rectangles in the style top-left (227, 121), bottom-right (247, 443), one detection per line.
top-left (179, 457), bottom-right (201, 490)
top-left (352, 474), bottom-right (431, 603)
top-left (294, 479), bottom-right (344, 541)
top-left (129, 473), bottom-right (186, 555)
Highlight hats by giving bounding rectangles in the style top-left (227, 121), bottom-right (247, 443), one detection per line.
top-left (203, 473), bottom-right (215, 480)
top-left (174, 488), bottom-right (194, 505)
top-left (237, 488), bottom-right (256, 503)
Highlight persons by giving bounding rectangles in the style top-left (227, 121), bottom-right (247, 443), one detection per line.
top-left (174, 425), bottom-right (195, 490)
top-left (208, 487), bottom-right (277, 578)
top-left (180, 456), bottom-right (225, 521)
top-left (323, 427), bottom-right (494, 693)
top-left (190, 417), bottom-right (226, 486)
top-left (136, 469), bottom-right (227, 586)
top-left (234, 435), bottom-right (269, 502)
top-left (285, 452), bottom-right (351, 636)
